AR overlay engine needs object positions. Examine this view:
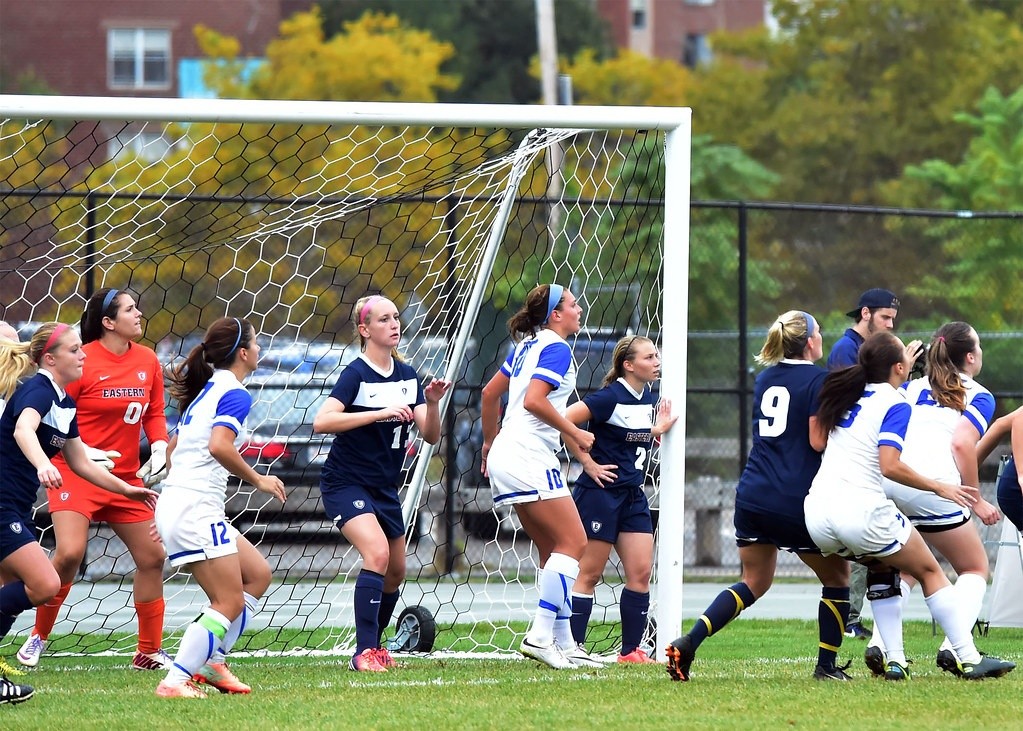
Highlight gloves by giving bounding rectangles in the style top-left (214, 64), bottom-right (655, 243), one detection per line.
top-left (138, 441), bottom-right (169, 487)
top-left (82, 441), bottom-right (123, 472)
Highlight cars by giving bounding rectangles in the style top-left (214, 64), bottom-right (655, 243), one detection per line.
top-left (154, 340), bottom-right (626, 544)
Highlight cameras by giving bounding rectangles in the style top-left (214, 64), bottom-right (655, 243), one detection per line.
top-left (912, 343), bottom-right (931, 371)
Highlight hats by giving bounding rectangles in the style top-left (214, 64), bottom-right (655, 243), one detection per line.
top-left (846, 288), bottom-right (900, 317)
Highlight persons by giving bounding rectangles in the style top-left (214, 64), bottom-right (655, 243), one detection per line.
top-left (665, 309), bottom-right (854, 681)
top-left (803, 330), bottom-right (1016, 682)
top-left (148, 317), bottom-right (288, 699)
top-left (0, 289), bottom-right (176, 707)
top-left (480, 284), bottom-right (606, 670)
top-left (565, 334), bottom-right (680, 669)
top-left (313, 295), bottom-right (453, 673)
top-left (823, 288), bottom-right (1023, 681)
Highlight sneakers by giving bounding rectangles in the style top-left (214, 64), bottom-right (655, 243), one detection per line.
top-left (193, 663), bottom-right (250, 694)
top-left (865, 639), bottom-right (888, 676)
top-left (17, 634), bottom-right (47, 667)
top-left (843, 617), bottom-right (872, 640)
top-left (156, 682), bottom-right (208, 699)
top-left (348, 649), bottom-right (388, 673)
top-left (374, 645), bottom-right (397, 667)
top-left (665, 636), bottom-right (695, 680)
top-left (520, 633), bottom-right (577, 670)
top-left (936, 642), bottom-right (963, 676)
top-left (961, 652), bottom-right (1015, 680)
top-left (560, 643), bottom-right (604, 669)
top-left (814, 660), bottom-right (855, 680)
top-left (618, 647), bottom-right (656, 664)
top-left (885, 659), bottom-right (914, 681)
top-left (0, 675), bottom-right (35, 704)
top-left (133, 648), bottom-right (174, 670)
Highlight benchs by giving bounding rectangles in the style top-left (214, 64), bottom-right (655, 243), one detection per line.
top-left (418, 480), bottom-right (736, 570)
